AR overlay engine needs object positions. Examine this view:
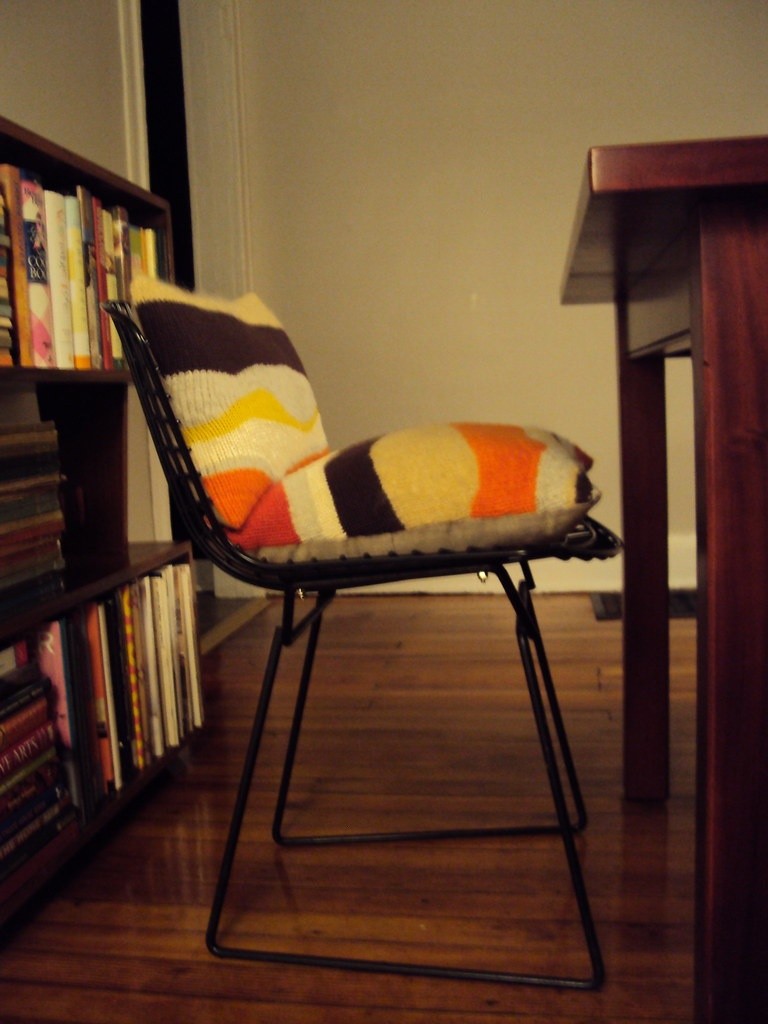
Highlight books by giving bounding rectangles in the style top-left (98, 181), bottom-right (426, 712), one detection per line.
top-left (0, 377), bottom-right (66, 615)
top-left (0, 562), bottom-right (205, 885)
top-left (0, 159), bottom-right (169, 370)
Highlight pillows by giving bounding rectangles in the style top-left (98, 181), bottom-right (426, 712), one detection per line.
top-left (225, 420), bottom-right (601, 564)
top-left (133, 290), bottom-right (331, 531)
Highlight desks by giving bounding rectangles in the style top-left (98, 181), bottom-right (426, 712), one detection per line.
top-left (558, 133), bottom-right (768, 1024)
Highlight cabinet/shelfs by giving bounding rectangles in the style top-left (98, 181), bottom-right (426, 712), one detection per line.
top-left (0, 117), bottom-right (202, 935)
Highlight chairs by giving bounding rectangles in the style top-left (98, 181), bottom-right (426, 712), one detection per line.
top-left (99, 301), bottom-right (609, 994)
